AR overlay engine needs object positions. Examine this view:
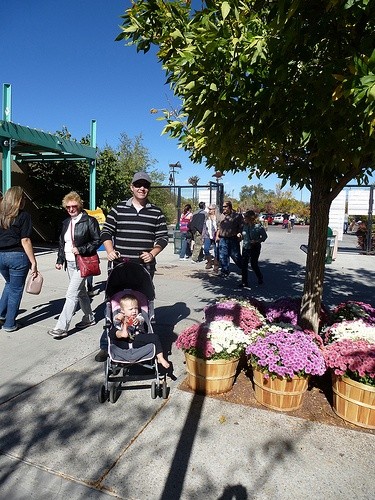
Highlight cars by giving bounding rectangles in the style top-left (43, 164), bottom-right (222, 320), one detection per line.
top-left (258, 213), bottom-right (306, 226)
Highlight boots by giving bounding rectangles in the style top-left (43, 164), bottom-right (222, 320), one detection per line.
top-left (213, 256), bottom-right (220, 276)
top-left (205, 253), bottom-right (212, 269)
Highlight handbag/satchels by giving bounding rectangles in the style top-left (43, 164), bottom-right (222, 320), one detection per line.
top-left (77, 254), bottom-right (101, 278)
top-left (187, 210), bottom-right (205, 235)
top-left (25, 270), bottom-right (43, 295)
top-left (180, 213), bottom-right (190, 231)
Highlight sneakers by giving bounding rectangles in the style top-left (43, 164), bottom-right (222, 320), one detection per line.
top-left (47, 329), bottom-right (68, 337)
top-left (75, 320), bottom-right (96, 329)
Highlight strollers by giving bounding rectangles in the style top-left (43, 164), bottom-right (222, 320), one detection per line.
top-left (96, 251), bottom-right (170, 403)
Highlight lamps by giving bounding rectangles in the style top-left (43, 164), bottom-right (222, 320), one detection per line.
top-left (3, 140), bottom-right (9, 147)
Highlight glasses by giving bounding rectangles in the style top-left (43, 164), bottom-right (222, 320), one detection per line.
top-left (66, 205), bottom-right (79, 209)
top-left (223, 206), bottom-right (228, 207)
top-left (209, 209), bottom-right (212, 210)
top-left (133, 182), bottom-right (150, 188)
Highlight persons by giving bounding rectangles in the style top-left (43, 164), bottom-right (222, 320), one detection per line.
top-left (47, 191), bottom-right (100, 337)
top-left (0, 187), bottom-right (38, 332)
top-left (282, 212), bottom-right (295, 229)
top-left (94, 172), bottom-right (169, 362)
top-left (180, 201), bottom-right (267, 288)
top-left (107, 292), bottom-right (169, 369)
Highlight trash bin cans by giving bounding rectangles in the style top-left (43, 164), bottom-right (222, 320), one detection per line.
top-left (173, 231), bottom-right (185, 254)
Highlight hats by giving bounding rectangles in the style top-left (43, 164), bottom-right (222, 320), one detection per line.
top-left (131, 172), bottom-right (152, 184)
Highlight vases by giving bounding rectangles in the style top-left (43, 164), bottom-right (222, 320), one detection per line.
top-left (184, 351), bottom-right (239, 394)
top-left (251, 367), bottom-right (308, 411)
top-left (331, 371), bottom-right (375, 429)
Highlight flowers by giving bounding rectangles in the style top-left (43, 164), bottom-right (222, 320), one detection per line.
top-left (176, 293), bottom-right (375, 385)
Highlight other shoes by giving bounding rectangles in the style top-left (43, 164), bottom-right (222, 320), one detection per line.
top-left (255, 281), bottom-right (264, 287)
top-left (191, 260), bottom-right (196, 263)
top-left (238, 283), bottom-right (248, 288)
top-left (87, 289), bottom-right (98, 296)
top-left (0, 319), bottom-right (21, 332)
top-left (95, 349), bottom-right (108, 362)
top-left (199, 258), bottom-right (207, 263)
top-left (185, 256), bottom-right (190, 260)
top-left (221, 272), bottom-right (229, 278)
top-left (179, 258), bottom-right (189, 261)
top-left (247, 262), bottom-right (253, 272)
